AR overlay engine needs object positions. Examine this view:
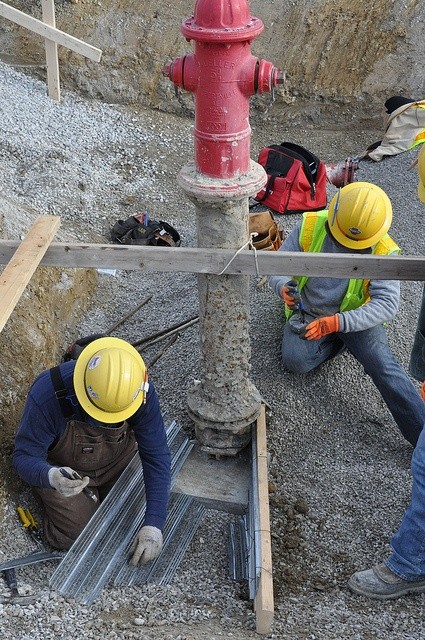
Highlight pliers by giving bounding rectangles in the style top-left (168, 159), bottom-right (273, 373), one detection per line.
top-left (59, 468), bottom-right (97, 503)
top-left (17, 507), bottom-right (45, 552)
top-left (287, 280), bottom-right (305, 324)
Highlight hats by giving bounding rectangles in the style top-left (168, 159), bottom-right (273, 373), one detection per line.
top-left (384, 96), bottom-right (415, 114)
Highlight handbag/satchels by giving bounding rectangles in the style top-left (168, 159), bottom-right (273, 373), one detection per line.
top-left (251, 142), bottom-right (327, 214)
top-left (249, 211), bottom-right (284, 251)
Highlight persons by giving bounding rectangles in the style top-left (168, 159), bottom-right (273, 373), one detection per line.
top-left (8, 335), bottom-right (172, 568)
top-left (346, 270), bottom-right (424, 601)
top-left (265, 181), bottom-right (425, 450)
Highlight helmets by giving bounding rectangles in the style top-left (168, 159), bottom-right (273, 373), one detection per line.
top-left (328, 182), bottom-right (393, 250)
top-left (417, 142), bottom-right (425, 203)
top-left (73, 337), bottom-right (149, 424)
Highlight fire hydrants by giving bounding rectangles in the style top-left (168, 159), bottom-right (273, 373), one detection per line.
top-left (161, 0), bottom-right (286, 180)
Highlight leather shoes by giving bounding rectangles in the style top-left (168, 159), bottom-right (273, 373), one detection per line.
top-left (348, 562), bottom-right (425, 599)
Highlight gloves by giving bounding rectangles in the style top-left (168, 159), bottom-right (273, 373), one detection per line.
top-left (129, 526), bottom-right (163, 566)
top-left (48, 466), bottom-right (90, 497)
top-left (299, 308), bottom-right (340, 341)
top-left (282, 280), bottom-right (302, 309)
